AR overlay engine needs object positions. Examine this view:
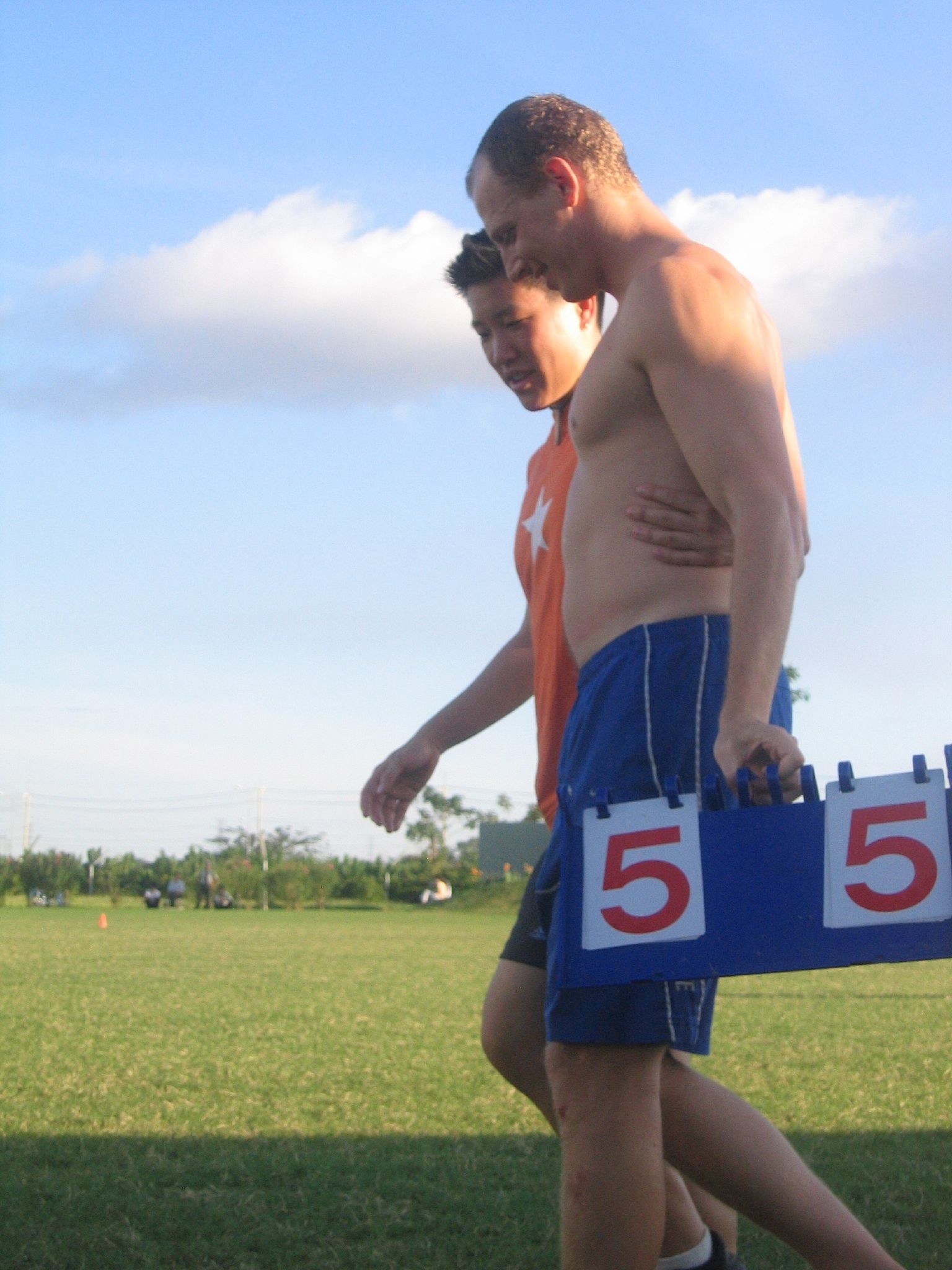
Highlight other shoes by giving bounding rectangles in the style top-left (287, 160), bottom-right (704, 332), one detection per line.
top-left (692, 1224), bottom-right (729, 1270)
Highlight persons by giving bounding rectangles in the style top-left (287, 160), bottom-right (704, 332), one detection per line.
top-left (215, 883), bottom-right (234, 909)
top-left (353, 93), bottom-right (913, 1270)
top-left (193, 861), bottom-right (220, 909)
top-left (167, 870), bottom-right (186, 907)
top-left (144, 882), bottom-right (162, 908)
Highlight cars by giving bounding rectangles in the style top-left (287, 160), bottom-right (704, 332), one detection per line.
top-left (392, 878), bottom-right (452, 905)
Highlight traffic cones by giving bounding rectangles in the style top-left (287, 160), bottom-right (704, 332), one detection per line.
top-left (98, 913), bottom-right (108, 929)
